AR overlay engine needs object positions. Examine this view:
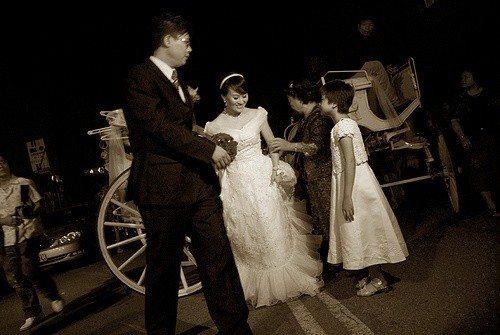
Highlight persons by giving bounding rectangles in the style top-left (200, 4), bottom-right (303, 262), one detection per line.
top-left (204, 71), bottom-right (410, 309)
top-left (0, 155), bottom-right (64, 331)
top-left (124, 7), bottom-right (256, 335)
top-left (451, 70), bottom-right (500, 218)
top-left (331, 12), bottom-right (413, 112)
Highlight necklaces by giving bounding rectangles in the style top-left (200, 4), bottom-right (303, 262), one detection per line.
top-left (224, 108), bottom-right (245, 119)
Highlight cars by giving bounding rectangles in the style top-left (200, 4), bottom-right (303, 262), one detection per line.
top-left (35, 201), bottom-right (97, 268)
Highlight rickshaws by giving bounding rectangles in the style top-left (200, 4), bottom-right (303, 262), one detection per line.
top-left (96, 55), bottom-right (466, 299)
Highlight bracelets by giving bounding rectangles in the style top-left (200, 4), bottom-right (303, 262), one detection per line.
top-left (459, 135), bottom-right (465, 139)
top-left (294, 142), bottom-right (298, 153)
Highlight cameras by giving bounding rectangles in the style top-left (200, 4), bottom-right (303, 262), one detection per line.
top-left (16, 185), bottom-right (33, 218)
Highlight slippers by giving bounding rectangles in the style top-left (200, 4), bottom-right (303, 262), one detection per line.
top-left (488, 206), bottom-right (500, 218)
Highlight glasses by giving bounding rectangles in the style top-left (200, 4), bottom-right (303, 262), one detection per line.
top-left (174, 38), bottom-right (190, 46)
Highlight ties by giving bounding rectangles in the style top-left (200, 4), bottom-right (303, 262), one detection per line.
top-left (172, 71), bottom-right (186, 102)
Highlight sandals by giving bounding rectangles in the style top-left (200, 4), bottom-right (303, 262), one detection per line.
top-left (357, 278), bottom-right (389, 296)
top-left (355, 275), bottom-right (368, 288)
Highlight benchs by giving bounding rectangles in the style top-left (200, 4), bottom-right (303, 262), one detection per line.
top-left (105, 126), bottom-right (134, 190)
top-left (316, 70), bottom-right (373, 112)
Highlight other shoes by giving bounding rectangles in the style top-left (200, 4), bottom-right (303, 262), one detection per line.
top-left (19, 313), bottom-right (43, 331)
top-left (52, 300), bottom-right (64, 312)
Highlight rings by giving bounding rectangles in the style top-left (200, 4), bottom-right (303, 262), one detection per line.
top-left (463, 143), bottom-right (466, 146)
